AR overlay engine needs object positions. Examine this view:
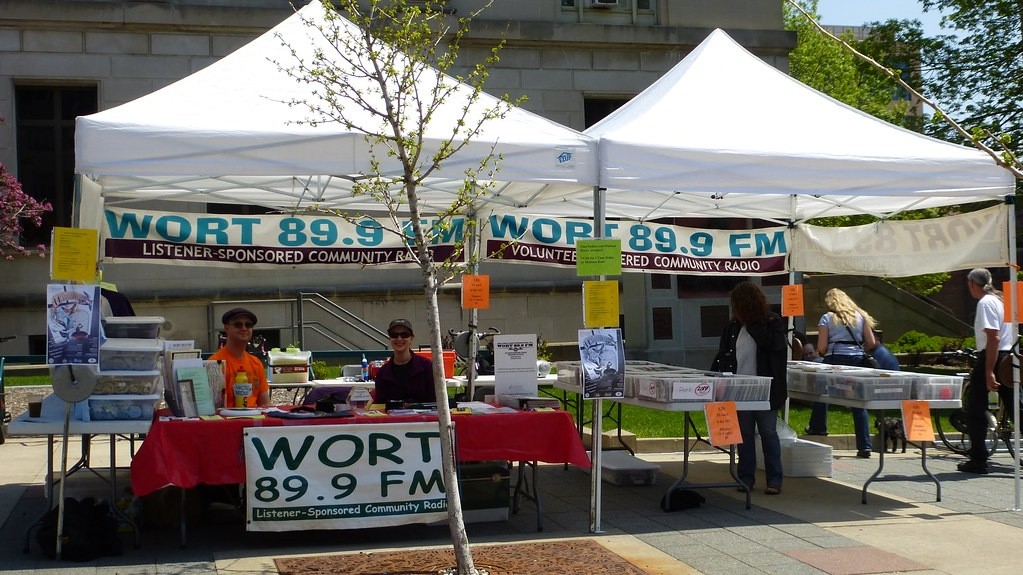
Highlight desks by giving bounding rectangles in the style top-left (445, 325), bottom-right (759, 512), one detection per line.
top-left (564, 389), bottom-right (767, 513)
top-left (790, 392), bottom-right (963, 504)
top-left (5, 395), bottom-right (570, 563)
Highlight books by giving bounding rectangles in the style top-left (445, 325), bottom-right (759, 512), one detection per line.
top-left (498, 394), bottom-right (561, 411)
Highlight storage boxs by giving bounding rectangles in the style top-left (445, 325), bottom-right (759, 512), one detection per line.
top-left (787, 362), bottom-right (965, 402)
top-left (74, 316), bottom-right (165, 419)
top-left (268, 351), bottom-right (311, 384)
top-left (555, 361), bottom-right (772, 404)
top-left (414, 351), bottom-right (455, 379)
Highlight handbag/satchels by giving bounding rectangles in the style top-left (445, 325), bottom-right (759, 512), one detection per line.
top-left (863, 353), bottom-right (881, 369)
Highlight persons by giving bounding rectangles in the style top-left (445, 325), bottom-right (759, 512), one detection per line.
top-left (207, 307), bottom-right (271, 415)
top-left (805, 287), bottom-right (875, 458)
top-left (862, 331), bottom-right (901, 371)
top-left (705, 282), bottom-right (788, 496)
top-left (797, 342), bottom-right (825, 407)
top-left (376, 319), bottom-right (436, 414)
top-left (957, 267), bottom-right (1023, 474)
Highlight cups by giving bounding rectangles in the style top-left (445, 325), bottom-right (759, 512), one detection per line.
top-left (27, 395), bottom-right (43, 418)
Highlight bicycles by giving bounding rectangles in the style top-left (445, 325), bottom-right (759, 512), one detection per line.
top-left (933, 346), bottom-right (1023, 468)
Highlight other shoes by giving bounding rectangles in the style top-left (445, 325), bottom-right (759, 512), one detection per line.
top-left (857, 451), bottom-right (871, 458)
top-left (805, 427), bottom-right (827, 436)
top-left (957, 462), bottom-right (989, 474)
top-left (765, 484), bottom-right (782, 495)
top-left (737, 484), bottom-right (753, 492)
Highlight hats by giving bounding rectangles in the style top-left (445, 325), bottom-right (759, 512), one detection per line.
top-left (387, 319), bottom-right (414, 335)
top-left (221, 307), bottom-right (257, 326)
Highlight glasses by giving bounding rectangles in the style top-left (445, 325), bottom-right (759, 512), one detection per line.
top-left (226, 322), bottom-right (254, 328)
top-left (388, 331), bottom-right (411, 338)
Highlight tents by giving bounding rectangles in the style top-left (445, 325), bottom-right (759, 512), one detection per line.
top-left (53, 0), bottom-right (1021, 563)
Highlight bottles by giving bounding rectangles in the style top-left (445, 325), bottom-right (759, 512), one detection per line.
top-left (361, 359), bottom-right (369, 382)
top-left (236, 365), bottom-right (248, 408)
top-left (989, 389), bottom-right (998, 409)
